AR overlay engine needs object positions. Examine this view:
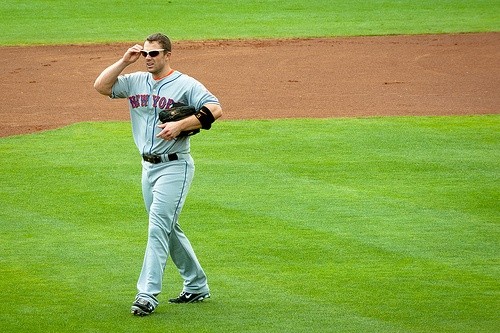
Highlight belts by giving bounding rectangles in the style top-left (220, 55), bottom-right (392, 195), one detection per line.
top-left (142, 152), bottom-right (178, 164)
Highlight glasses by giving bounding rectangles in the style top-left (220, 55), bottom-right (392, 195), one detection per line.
top-left (140, 49), bottom-right (170, 57)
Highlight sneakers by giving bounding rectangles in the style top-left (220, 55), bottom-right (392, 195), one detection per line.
top-left (168, 292), bottom-right (211, 304)
top-left (130, 298), bottom-right (155, 316)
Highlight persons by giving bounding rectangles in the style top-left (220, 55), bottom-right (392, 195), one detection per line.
top-left (93, 33), bottom-right (223, 316)
